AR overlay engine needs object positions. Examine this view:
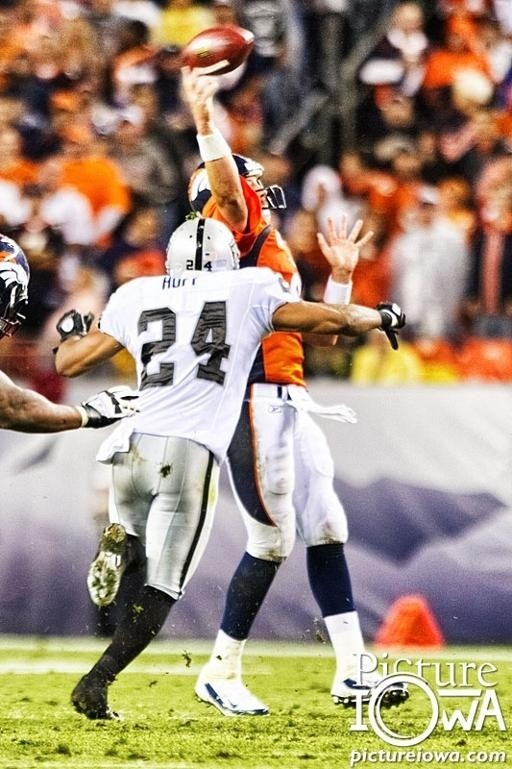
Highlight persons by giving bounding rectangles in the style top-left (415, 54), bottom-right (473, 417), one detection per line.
top-left (183, 74), bottom-right (411, 716)
top-left (49, 216), bottom-right (406, 718)
top-left (1, 0), bottom-right (510, 342)
top-left (1, 233), bottom-right (141, 435)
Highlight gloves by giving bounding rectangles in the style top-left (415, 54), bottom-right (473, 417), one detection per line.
top-left (80, 384), bottom-right (141, 427)
top-left (376, 299), bottom-right (407, 351)
top-left (56, 310), bottom-right (94, 341)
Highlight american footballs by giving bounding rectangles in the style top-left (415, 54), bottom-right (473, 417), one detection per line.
top-left (181, 25), bottom-right (255, 75)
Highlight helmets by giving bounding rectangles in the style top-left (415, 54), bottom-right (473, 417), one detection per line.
top-left (0, 233), bottom-right (30, 340)
top-left (166, 215), bottom-right (241, 276)
top-left (188, 155), bottom-right (264, 220)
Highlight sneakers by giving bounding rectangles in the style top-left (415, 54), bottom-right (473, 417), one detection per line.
top-left (193, 664), bottom-right (271, 718)
top-left (86, 523), bottom-right (131, 607)
top-left (329, 671), bottom-right (408, 709)
top-left (69, 677), bottom-right (125, 726)
